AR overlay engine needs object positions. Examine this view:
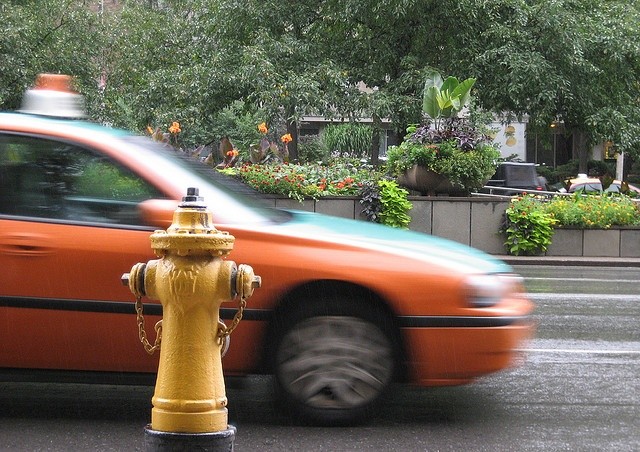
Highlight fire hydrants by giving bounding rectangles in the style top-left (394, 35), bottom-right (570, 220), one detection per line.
top-left (121, 186), bottom-right (262, 452)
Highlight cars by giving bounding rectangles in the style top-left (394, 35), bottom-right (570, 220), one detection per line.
top-left (556, 177), bottom-right (639, 198)
top-left (0, 110), bottom-right (536, 426)
